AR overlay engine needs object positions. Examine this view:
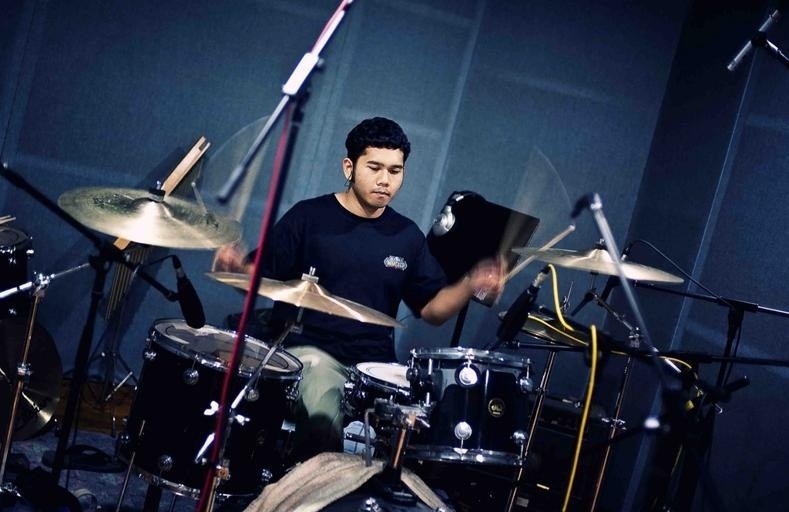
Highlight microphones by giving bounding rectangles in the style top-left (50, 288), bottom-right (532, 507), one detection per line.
top-left (496, 264), bottom-right (549, 337)
top-left (170, 253), bottom-right (207, 328)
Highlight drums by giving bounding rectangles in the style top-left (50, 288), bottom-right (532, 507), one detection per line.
top-left (406, 346), bottom-right (529, 473)
top-left (0, 223), bottom-right (34, 322)
top-left (1, 319), bottom-right (62, 438)
top-left (340, 362), bottom-right (411, 463)
top-left (124, 319), bottom-right (302, 499)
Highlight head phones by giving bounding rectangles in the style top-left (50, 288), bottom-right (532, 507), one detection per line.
top-left (432, 190), bottom-right (486, 236)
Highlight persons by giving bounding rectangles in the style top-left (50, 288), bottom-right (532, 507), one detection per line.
top-left (209, 115), bottom-right (503, 456)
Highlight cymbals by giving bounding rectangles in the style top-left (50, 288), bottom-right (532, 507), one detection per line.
top-left (58, 187), bottom-right (244, 250)
top-left (497, 310), bottom-right (627, 348)
top-left (206, 272), bottom-right (403, 329)
top-left (509, 247), bottom-right (685, 285)
